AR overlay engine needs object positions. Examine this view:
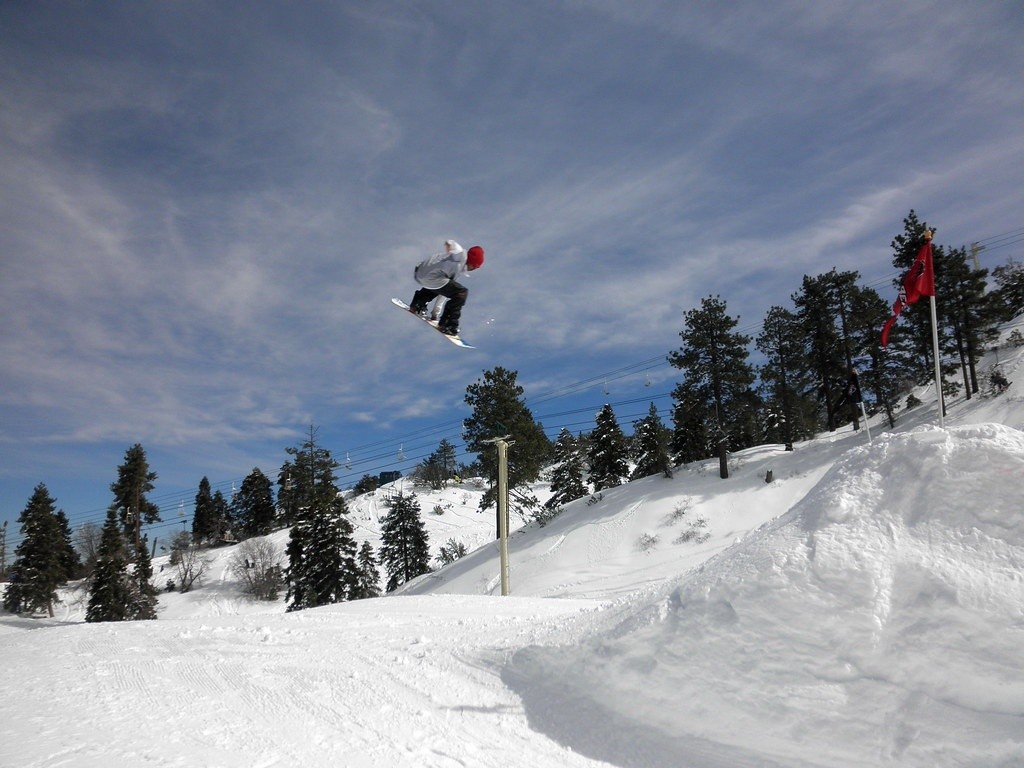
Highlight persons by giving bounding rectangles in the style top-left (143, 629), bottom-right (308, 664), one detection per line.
top-left (405, 240), bottom-right (484, 334)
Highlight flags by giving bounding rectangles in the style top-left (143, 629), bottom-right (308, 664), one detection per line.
top-left (881, 238), bottom-right (935, 350)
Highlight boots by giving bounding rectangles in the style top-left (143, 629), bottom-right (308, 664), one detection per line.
top-left (411, 290), bottom-right (430, 310)
top-left (438, 307), bottom-right (460, 333)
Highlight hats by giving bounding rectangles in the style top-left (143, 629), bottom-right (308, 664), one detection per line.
top-left (468, 246), bottom-right (484, 268)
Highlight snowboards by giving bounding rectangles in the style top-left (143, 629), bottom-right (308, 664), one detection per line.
top-left (391, 297), bottom-right (476, 349)
